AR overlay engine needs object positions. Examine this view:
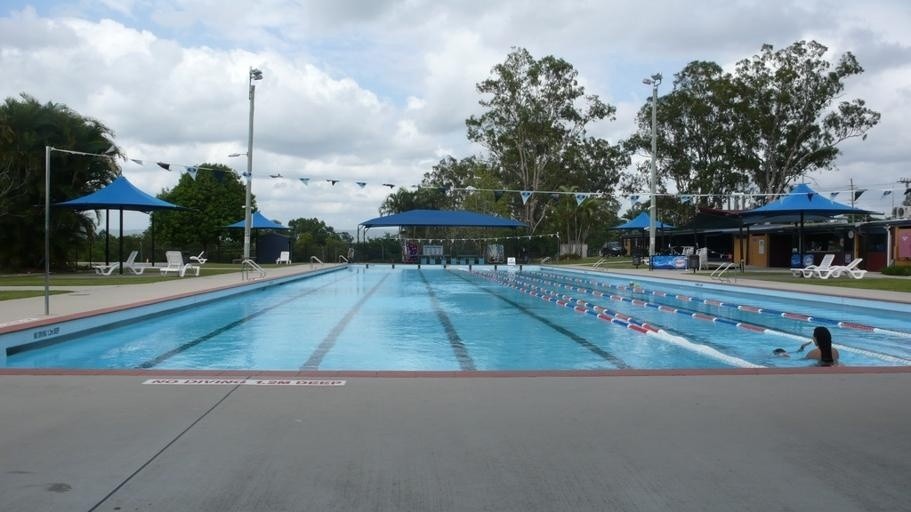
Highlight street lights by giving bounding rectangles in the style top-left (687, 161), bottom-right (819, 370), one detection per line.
top-left (642, 72), bottom-right (663, 258)
top-left (243, 66), bottom-right (264, 259)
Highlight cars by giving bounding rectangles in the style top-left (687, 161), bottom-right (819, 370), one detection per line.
top-left (599, 242), bottom-right (627, 257)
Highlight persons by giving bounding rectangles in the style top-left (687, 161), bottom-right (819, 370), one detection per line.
top-left (769, 340), bottom-right (812, 358)
top-left (800, 325), bottom-right (839, 366)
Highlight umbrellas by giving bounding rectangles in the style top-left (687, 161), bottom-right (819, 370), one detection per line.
top-left (741, 183), bottom-right (885, 267)
top-left (221, 211), bottom-right (294, 263)
top-left (606, 211), bottom-right (672, 264)
top-left (49, 175), bottom-right (188, 273)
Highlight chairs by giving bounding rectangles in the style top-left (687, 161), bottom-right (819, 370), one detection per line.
top-left (160, 251), bottom-right (200, 278)
top-left (682, 245), bottom-right (709, 271)
top-left (92, 250), bottom-right (144, 276)
top-left (804, 258), bottom-right (867, 279)
top-left (189, 250), bottom-right (207, 264)
top-left (275, 251), bottom-right (291, 265)
top-left (789, 254), bottom-right (836, 278)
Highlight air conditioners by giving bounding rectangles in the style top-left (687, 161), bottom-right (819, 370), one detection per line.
top-left (892, 206), bottom-right (906, 218)
